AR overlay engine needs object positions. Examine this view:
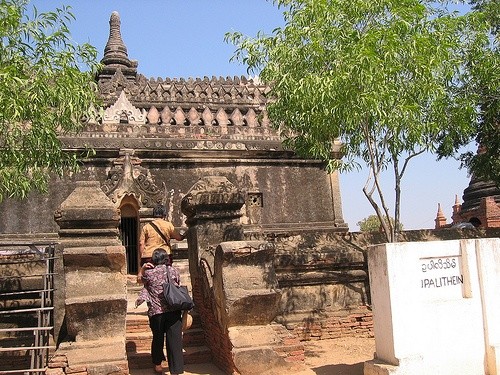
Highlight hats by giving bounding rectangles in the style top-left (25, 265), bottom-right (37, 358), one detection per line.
top-left (182, 310), bottom-right (193, 331)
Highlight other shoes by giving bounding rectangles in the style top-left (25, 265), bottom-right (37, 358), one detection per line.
top-left (153, 364), bottom-right (163, 374)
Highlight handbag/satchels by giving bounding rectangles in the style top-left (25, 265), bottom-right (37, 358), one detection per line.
top-left (162, 265), bottom-right (195, 310)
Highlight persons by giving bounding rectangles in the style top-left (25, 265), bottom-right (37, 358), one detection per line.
top-left (135, 248), bottom-right (188, 375)
top-left (140, 205), bottom-right (190, 270)
top-left (450, 217), bottom-right (489, 238)
